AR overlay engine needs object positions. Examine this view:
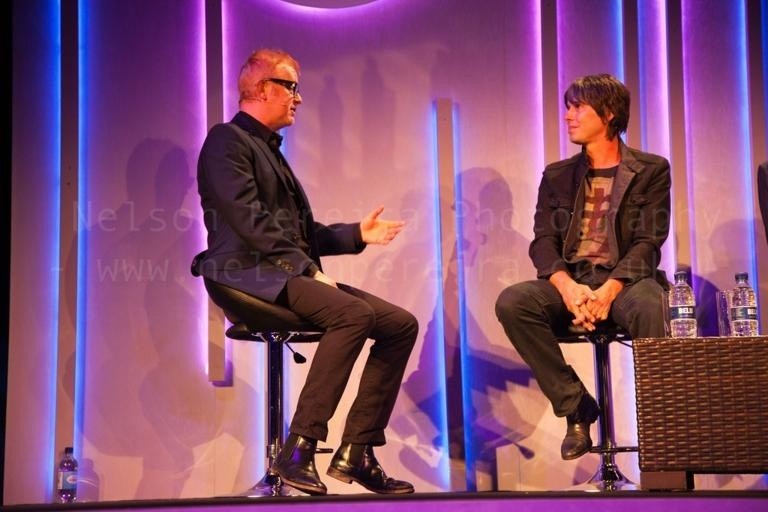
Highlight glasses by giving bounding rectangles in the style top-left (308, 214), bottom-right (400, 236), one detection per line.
top-left (254, 77), bottom-right (300, 96)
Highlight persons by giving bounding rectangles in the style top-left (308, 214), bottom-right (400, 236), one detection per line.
top-left (494, 71), bottom-right (674, 460)
top-left (195, 47), bottom-right (422, 496)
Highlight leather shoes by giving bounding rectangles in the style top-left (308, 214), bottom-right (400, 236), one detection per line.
top-left (561, 391), bottom-right (601, 461)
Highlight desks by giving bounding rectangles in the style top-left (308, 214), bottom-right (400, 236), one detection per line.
top-left (633, 336), bottom-right (768, 490)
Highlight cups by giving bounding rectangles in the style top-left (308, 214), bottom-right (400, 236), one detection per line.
top-left (716, 290), bottom-right (735, 338)
top-left (662, 291), bottom-right (674, 339)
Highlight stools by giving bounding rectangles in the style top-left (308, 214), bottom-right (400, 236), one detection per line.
top-left (555, 271), bottom-right (668, 492)
top-left (204, 276), bottom-right (333, 497)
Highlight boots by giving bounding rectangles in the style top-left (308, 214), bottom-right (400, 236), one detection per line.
top-left (271, 432), bottom-right (327, 496)
top-left (326, 440), bottom-right (415, 494)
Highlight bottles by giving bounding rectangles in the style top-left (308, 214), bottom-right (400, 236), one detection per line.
top-left (730, 272), bottom-right (759, 337)
top-left (668, 271), bottom-right (697, 339)
top-left (56, 447), bottom-right (78, 504)
